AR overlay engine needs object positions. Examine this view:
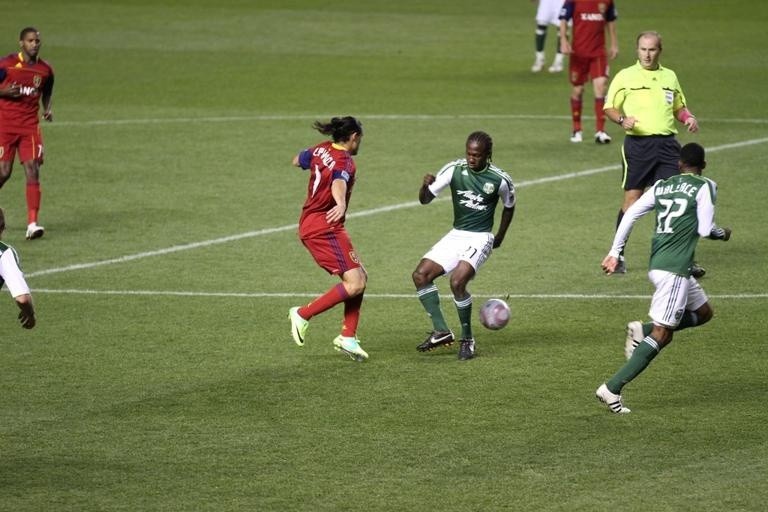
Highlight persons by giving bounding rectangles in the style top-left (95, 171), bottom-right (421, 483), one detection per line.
top-left (529, 0), bottom-right (585, 76)
top-left (595, 143), bottom-right (732, 415)
top-left (558, 0), bottom-right (620, 145)
top-left (601, 27), bottom-right (709, 279)
top-left (1, 27), bottom-right (55, 241)
top-left (411, 131), bottom-right (516, 362)
top-left (0, 206), bottom-right (37, 331)
top-left (288, 114), bottom-right (372, 363)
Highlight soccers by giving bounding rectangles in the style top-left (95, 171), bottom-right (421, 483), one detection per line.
top-left (478, 298), bottom-right (511, 331)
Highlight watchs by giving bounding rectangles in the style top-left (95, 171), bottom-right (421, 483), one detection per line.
top-left (617, 115), bottom-right (625, 126)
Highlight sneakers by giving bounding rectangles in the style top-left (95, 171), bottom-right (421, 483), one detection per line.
top-left (333, 334), bottom-right (369, 363)
top-left (626, 321), bottom-right (644, 361)
top-left (548, 58), bottom-right (565, 73)
top-left (415, 330), bottom-right (456, 353)
top-left (25, 223), bottom-right (44, 241)
top-left (593, 130), bottom-right (613, 144)
top-left (460, 337), bottom-right (477, 361)
top-left (287, 306), bottom-right (311, 347)
top-left (531, 54), bottom-right (547, 73)
top-left (595, 382), bottom-right (631, 415)
top-left (570, 129), bottom-right (586, 142)
top-left (690, 263), bottom-right (709, 277)
